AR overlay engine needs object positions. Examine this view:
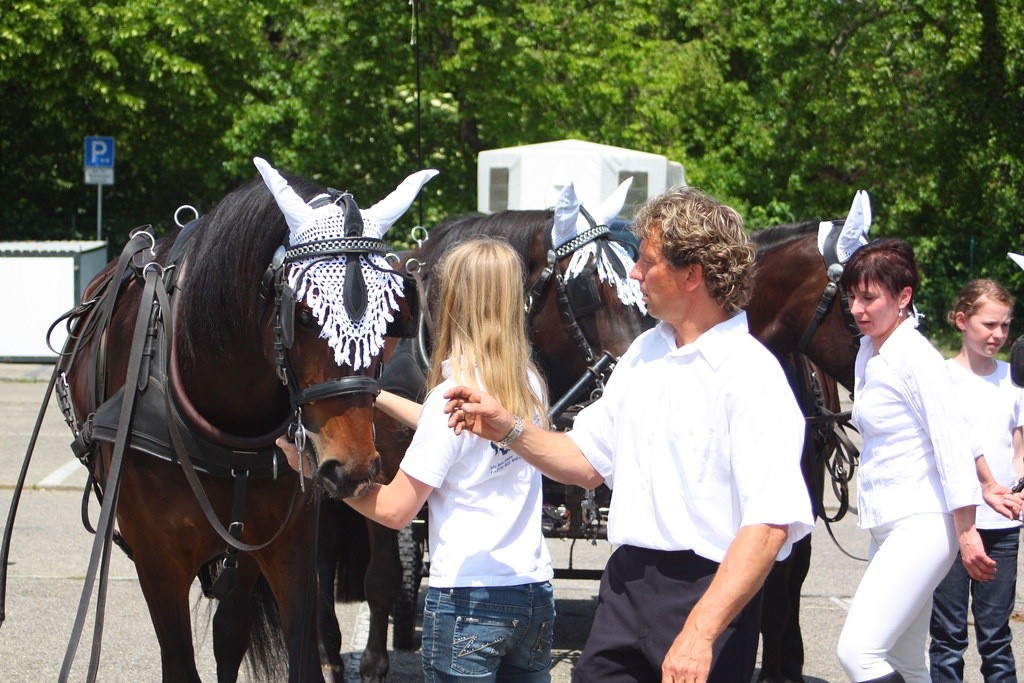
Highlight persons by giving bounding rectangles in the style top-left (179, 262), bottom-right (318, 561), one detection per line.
top-left (835, 237), bottom-right (998, 683)
top-left (275, 233), bottom-right (550, 683)
top-left (444, 186), bottom-right (816, 683)
top-left (929, 277), bottom-right (1024, 683)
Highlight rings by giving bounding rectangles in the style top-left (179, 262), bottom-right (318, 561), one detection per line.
top-left (461, 409), bottom-right (466, 419)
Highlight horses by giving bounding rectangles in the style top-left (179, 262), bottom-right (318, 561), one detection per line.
top-left (743, 190), bottom-right (874, 683)
top-left (67, 156), bottom-right (439, 683)
top-left (312, 174), bottom-right (656, 683)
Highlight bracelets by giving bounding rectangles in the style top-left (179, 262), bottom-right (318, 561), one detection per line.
top-left (493, 415), bottom-right (524, 449)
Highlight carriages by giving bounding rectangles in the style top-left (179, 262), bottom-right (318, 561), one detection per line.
top-left (1, 139), bottom-right (1023, 683)
